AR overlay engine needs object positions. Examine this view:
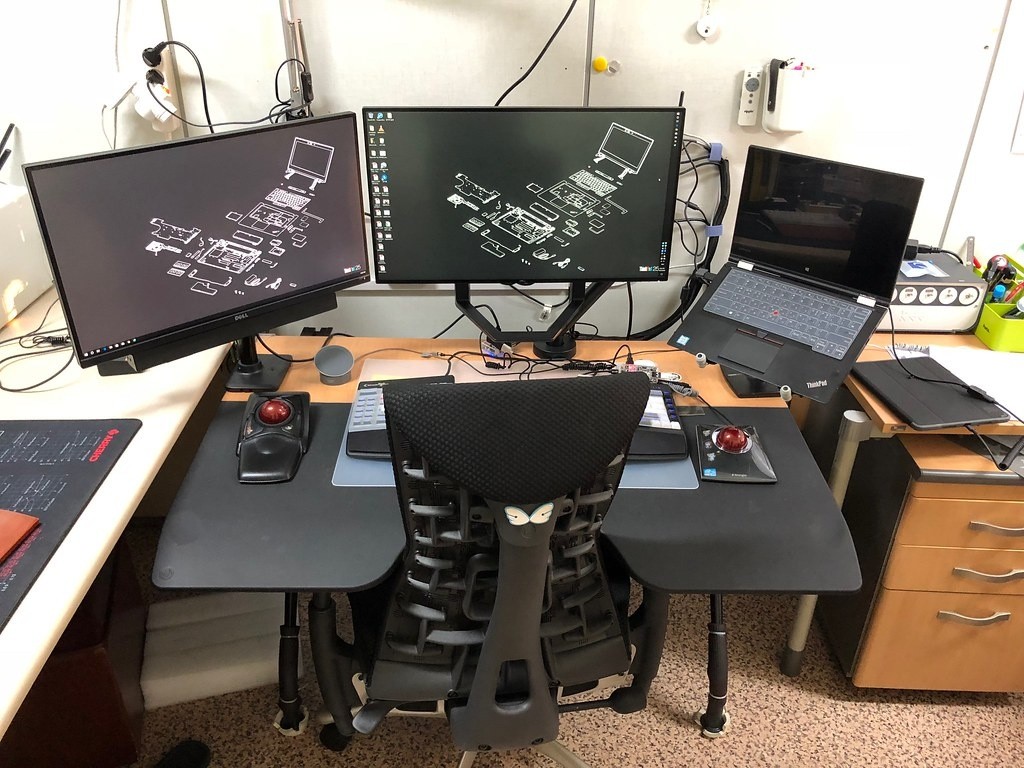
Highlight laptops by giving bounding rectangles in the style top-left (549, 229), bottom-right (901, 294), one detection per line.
top-left (666, 145), bottom-right (924, 403)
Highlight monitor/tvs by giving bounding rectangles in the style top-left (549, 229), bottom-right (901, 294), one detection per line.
top-left (363, 106), bottom-right (686, 363)
top-left (21, 111), bottom-right (371, 392)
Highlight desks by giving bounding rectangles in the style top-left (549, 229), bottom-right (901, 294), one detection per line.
top-left (134, 333), bottom-right (866, 739)
top-left (0, 302), bottom-right (239, 768)
top-left (781, 329), bottom-right (1024, 677)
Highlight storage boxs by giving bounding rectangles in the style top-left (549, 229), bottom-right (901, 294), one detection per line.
top-left (975, 303), bottom-right (1024, 353)
top-left (0, 530), bottom-right (147, 768)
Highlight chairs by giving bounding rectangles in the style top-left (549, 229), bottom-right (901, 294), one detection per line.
top-left (364, 370), bottom-right (653, 768)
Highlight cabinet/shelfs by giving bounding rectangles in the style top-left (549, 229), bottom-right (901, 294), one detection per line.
top-left (820, 434), bottom-right (1024, 694)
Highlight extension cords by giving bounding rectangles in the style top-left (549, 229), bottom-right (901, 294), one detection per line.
top-left (126, 59), bottom-right (172, 120)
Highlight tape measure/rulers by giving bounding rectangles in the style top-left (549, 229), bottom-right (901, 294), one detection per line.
top-left (966, 236), bottom-right (974, 272)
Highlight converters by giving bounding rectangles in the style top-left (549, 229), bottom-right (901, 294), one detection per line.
top-left (903, 239), bottom-right (918, 260)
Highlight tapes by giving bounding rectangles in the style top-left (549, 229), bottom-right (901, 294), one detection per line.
top-left (991, 256), bottom-right (1008, 270)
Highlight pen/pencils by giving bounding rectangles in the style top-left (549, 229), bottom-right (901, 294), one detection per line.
top-left (795, 63), bottom-right (816, 70)
top-left (972, 254), bottom-right (1024, 317)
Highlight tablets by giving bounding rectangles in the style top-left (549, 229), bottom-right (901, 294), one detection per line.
top-left (851, 356), bottom-right (1011, 431)
